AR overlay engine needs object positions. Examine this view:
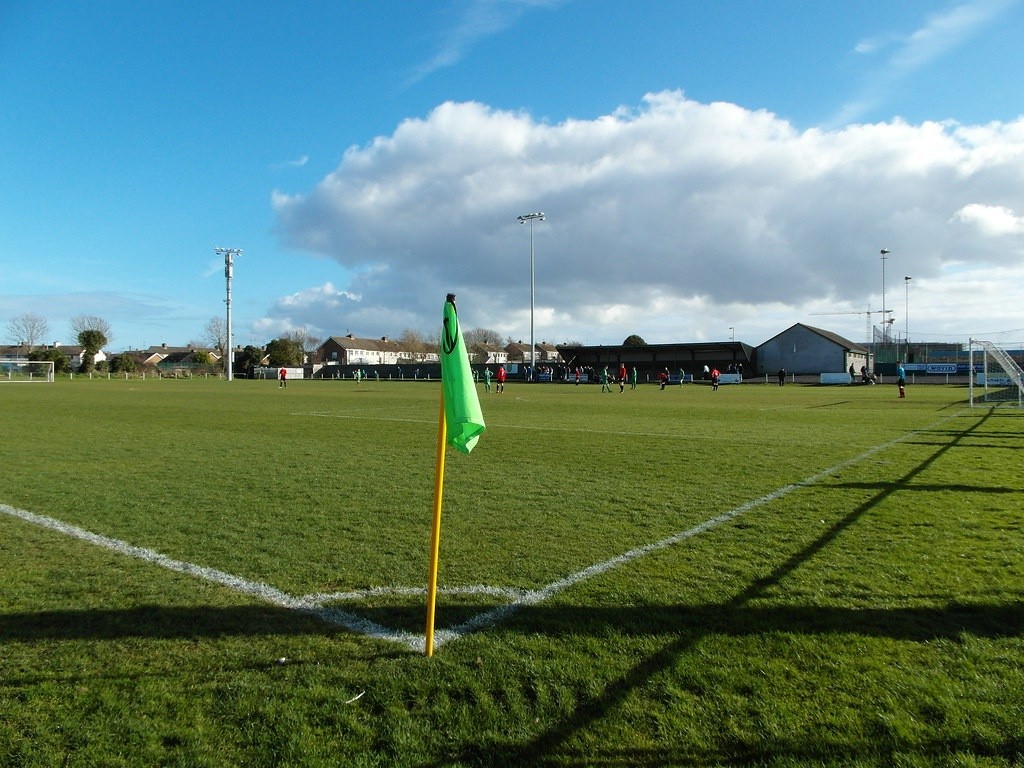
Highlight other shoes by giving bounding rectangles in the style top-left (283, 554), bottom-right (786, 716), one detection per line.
top-left (501, 391), bottom-right (503, 393)
top-left (278, 387), bottom-right (282, 388)
top-left (496, 391), bottom-right (499, 393)
top-left (660, 388), bottom-right (663, 390)
top-left (609, 391), bottom-right (612, 392)
top-left (602, 391), bottom-right (605, 393)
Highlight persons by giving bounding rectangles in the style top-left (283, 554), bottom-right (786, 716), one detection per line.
top-left (576, 365), bottom-right (615, 393)
top-left (861, 365), bottom-right (876, 386)
top-left (522, 365), bottom-right (567, 383)
top-left (657, 367), bottom-right (670, 390)
top-left (481, 367), bottom-right (494, 393)
top-left (849, 363), bottom-right (855, 380)
top-left (354, 369), bottom-right (365, 384)
top-left (702, 363), bottom-right (744, 391)
top-left (631, 367), bottom-right (637, 389)
top-left (618, 363), bottom-right (626, 393)
top-left (279, 367), bottom-right (287, 388)
top-left (374, 370), bottom-right (377, 377)
top-left (679, 368), bottom-right (685, 387)
top-left (896, 361), bottom-right (905, 398)
top-left (496, 366), bottom-right (506, 393)
top-left (778, 368), bottom-right (786, 386)
top-left (336, 370), bottom-right (340, 378)
top-left (397, 367), bottom-right (402, 377)
top-left (414, 369), bottom-right (420, 376)
